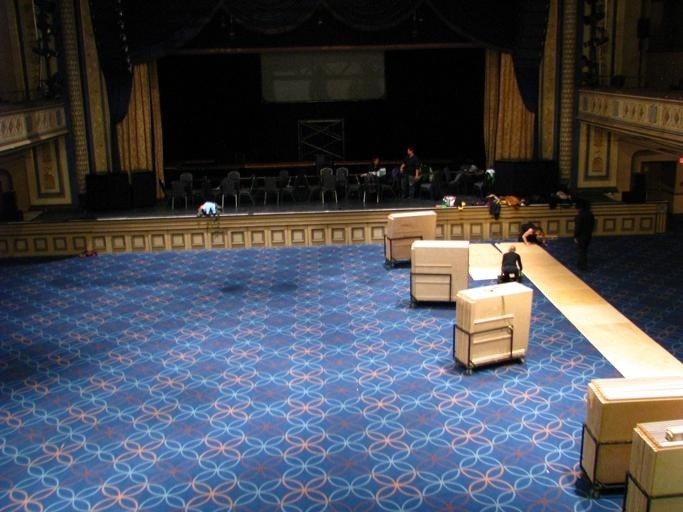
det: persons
[365,156,381,177]
[516,222,542,245]
[573,202,594,267]
[500,246,522,282]
[398,147,422,202]
